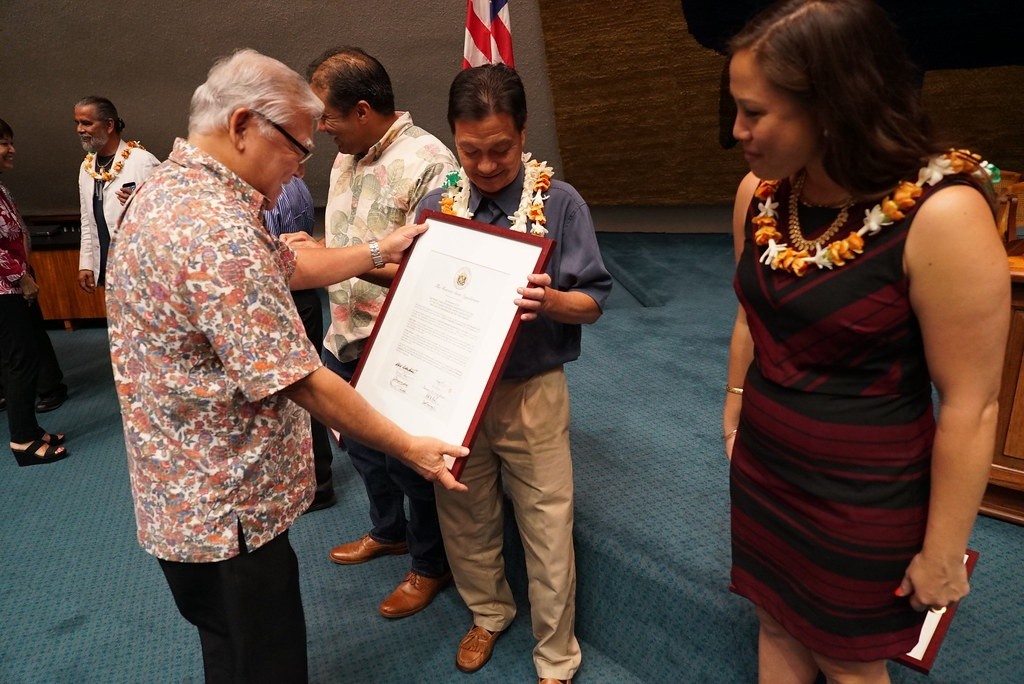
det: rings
[927,606,947,614]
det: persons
[259,174,337,514]
[103,48,470,684]
[411,63,614,684]
[721,2,1011,684]
[277,45,462,618]
[74,95,163,293]
[0,116,70,466]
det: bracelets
[721,430,738,438]
[725,384,744,394]
[368,239,384,270]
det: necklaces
[749,146,1000,280]
[437,150,555,237]
[81,142,147,182]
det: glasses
[252,109,316,165]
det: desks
[30,227,107,332]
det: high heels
[34,425,65,446]
[11,439,68,466]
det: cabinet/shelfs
[977,237,1024,523]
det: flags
[461,0,512,70]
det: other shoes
[35,391,68,413]
[304,491,338,515]
[0,398,7,411]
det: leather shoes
[456,623,500,672]
[329,532,408,564]
[538,672,571,684]
[380,563,452,618]
